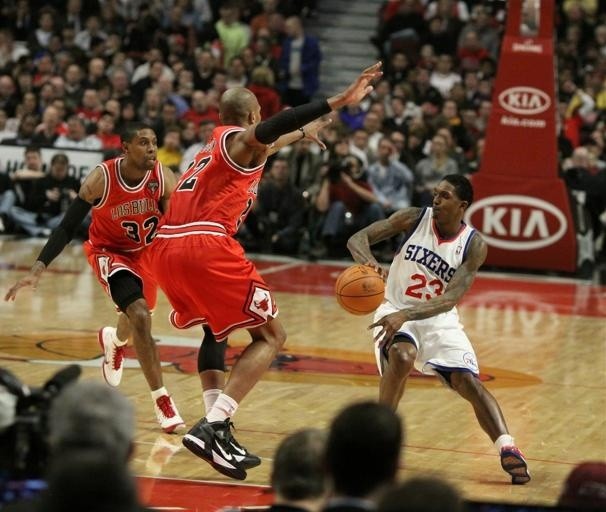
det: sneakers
[151,394,185,434]
[98,324,129,387]
[498,442,533,485]
[181,413,249,480]
[227,435,264,470]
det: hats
[50,153,69,167]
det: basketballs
[335,264,384,316]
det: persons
[0,1,606,281]
[369,474,463,510]
[343,170,529,489]
[263,427,333,510]
[553,459,606,512]
[145,58,385,482]
[38,379,149,509]
[316,399,404,510]
[0,120,191,434]
